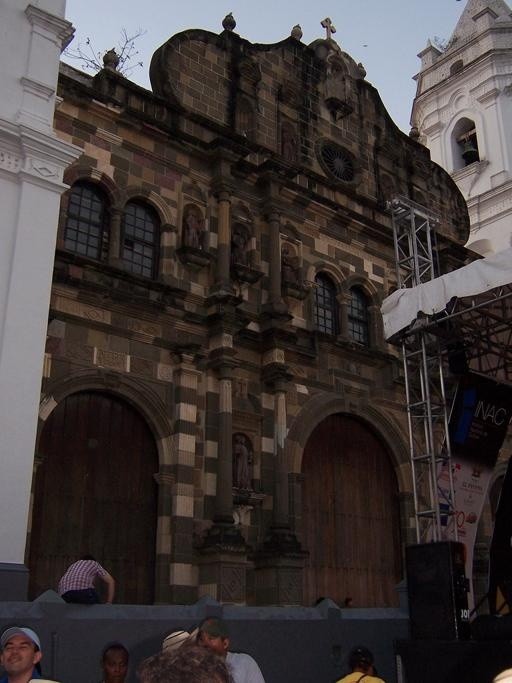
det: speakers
[405,540,471,641]
[440,369,512,468]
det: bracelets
[106,600,113,604]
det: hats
[0,626,41,649]
[201,619,226,637]
[348,646,374,666]
[162,627,199,649]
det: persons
[198,617,265,683]
[232,434,252,490]
[0,627,61,683]
[100,643,129,683]
[58,556,116,605]
[336,646,386,683]
